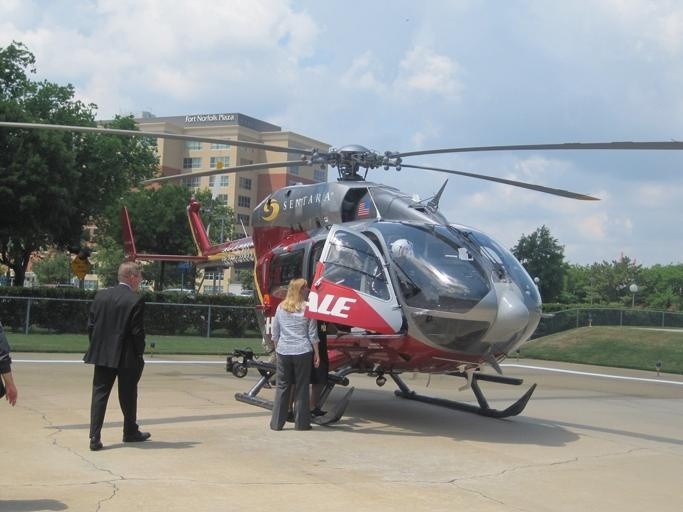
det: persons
[285,320,349,423]
[82,261,149,450]
[269,277,319,432]
[0,319,17,408]
[363,237,417,335]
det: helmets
[391,238,414,258]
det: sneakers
[286,407,328,423]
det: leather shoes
[88,436,105,451]
[121,430,152,443]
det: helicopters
[1,120,682,429]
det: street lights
[629,283,639,310]
[534,276,540,286]
[64,250,72,285]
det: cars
[35,284,261,332]
